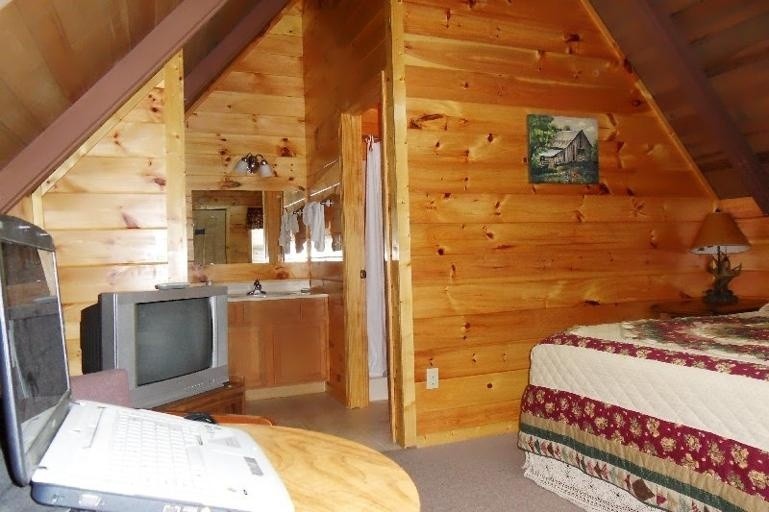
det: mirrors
[191,189,285,265]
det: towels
[276,184,342,253]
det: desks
[216,403,420,512]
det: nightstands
[654,298,767,315]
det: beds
[519,303,769,512]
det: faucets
[246,280,267,297]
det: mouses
[185,411,215,424]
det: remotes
[155,282,191,289]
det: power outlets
[427,366,439,390]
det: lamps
[235,153,274,178]
[689,208,748,304]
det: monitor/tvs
[79,285,231,409]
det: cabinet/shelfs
[228,296,330,400]
[149,375,246,420]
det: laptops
[0,212,296,512]
